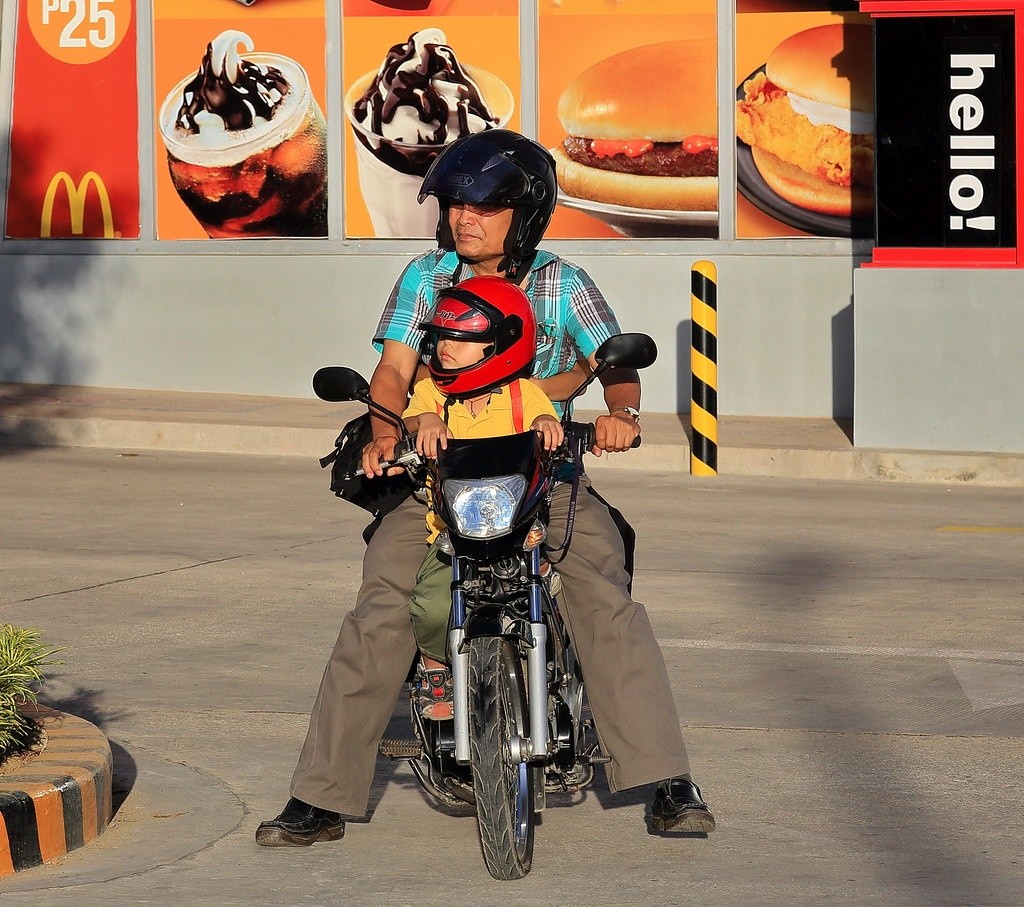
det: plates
[736,65,875,239]
[557,186,719,239]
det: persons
[399,275,564,721]
[255,129,715,845]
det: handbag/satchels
[319,412,427,517]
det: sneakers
[415,657,454,720]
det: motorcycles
[312,332,666,881]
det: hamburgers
[733,24,877,216]
[553,38,723,214]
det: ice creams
[164,29,304,165]
[354,27,499,238]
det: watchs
[610,406,641,425]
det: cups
[344,65,513,240]
[159,51,327,238]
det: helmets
[416,129,557,264]
[417,276,537,401]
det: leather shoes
[256,797,344,848]
[653,779,715,833]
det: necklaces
[466,394,491,419]
